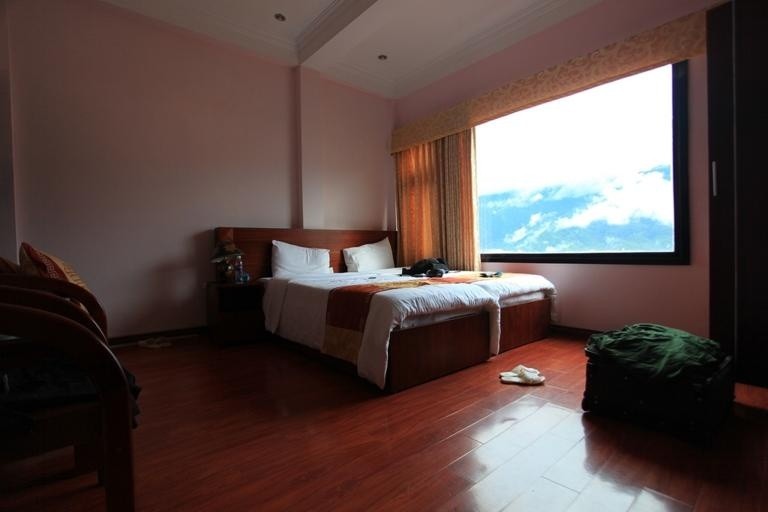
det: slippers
[499,365,540,377]
[501,369,545,384]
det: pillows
[0,242,91,294]
[343,236,395,272]
[272,239,330,278]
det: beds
[214,227,558,395]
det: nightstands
[207,282,263,349]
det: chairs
[0,278,142,512]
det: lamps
[209,240,245,282]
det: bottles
[235,255,242,282]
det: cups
[239,273,249,282]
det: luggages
[582,323,735,433]
